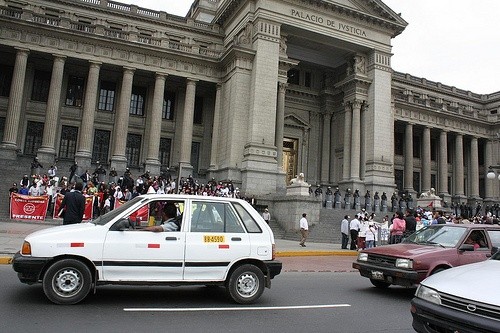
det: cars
[409,246,500,333]
[11,192,283,305]
[352,224,500,301]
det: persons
[134,170,257,219]
[8,183,19,220]
[354,189,500,250]
[349,214,360,250]
[334,186,341,208]
[309,187,314,194]
[298,213,308,247]
[324,186,332,207]
[343,188,351,209]
[314,185,323,196]
[20,157,134,217]
[262,208,270,226]
[340,215,349,249]
[57,183,86,226]
[140,204,180,232]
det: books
[57,209,65,218]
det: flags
[9,191,49,222]
[114,197,150,223]
[52,193,95,220]
